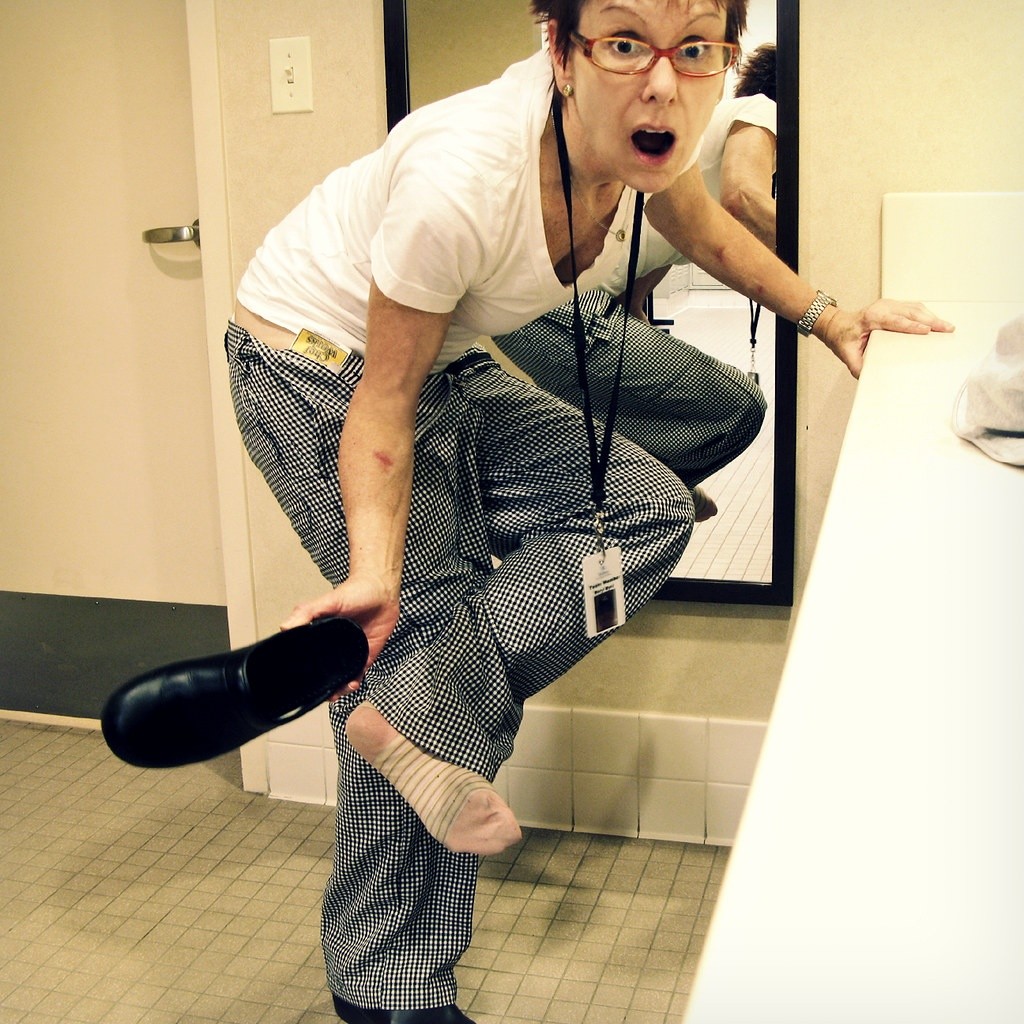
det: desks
[678,304,1024,1023]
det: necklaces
[571,175,633,242]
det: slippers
[333,993,477,1024]
[101,617,370,769]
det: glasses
[566,30,738,77]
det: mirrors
[379,0,799,610]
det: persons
[491,41,780,525]
[224,1,952,1023]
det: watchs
[796,290,837,337]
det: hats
[952,314,1024,467]
